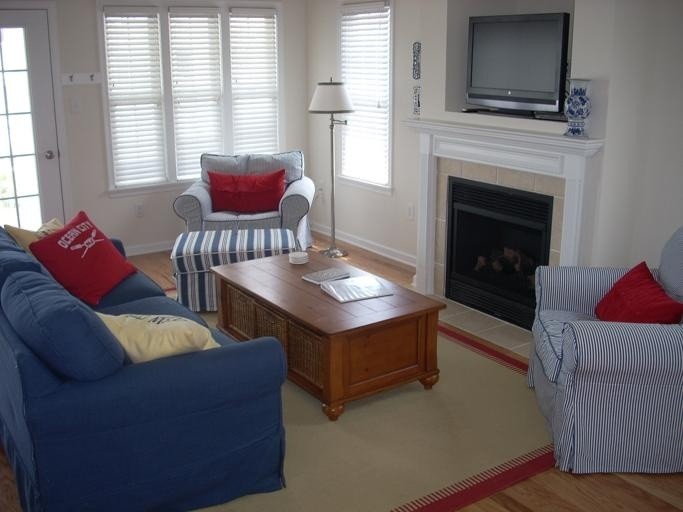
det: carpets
[165,285,558,511]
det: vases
[563,78,592,140]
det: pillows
[3,210,139,306]
[595,261,682,323]
[94,311,222,364]
[207,168,285,215]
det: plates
[289,251,309,265]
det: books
[318,272,393,304]
[300,266,348,286]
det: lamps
[308,77,356,259]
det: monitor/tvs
[462,11,572,122]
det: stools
[169,228,298,312]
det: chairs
[173,152,315,252]
[530,227,683,476]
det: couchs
[0,227,288,511]
